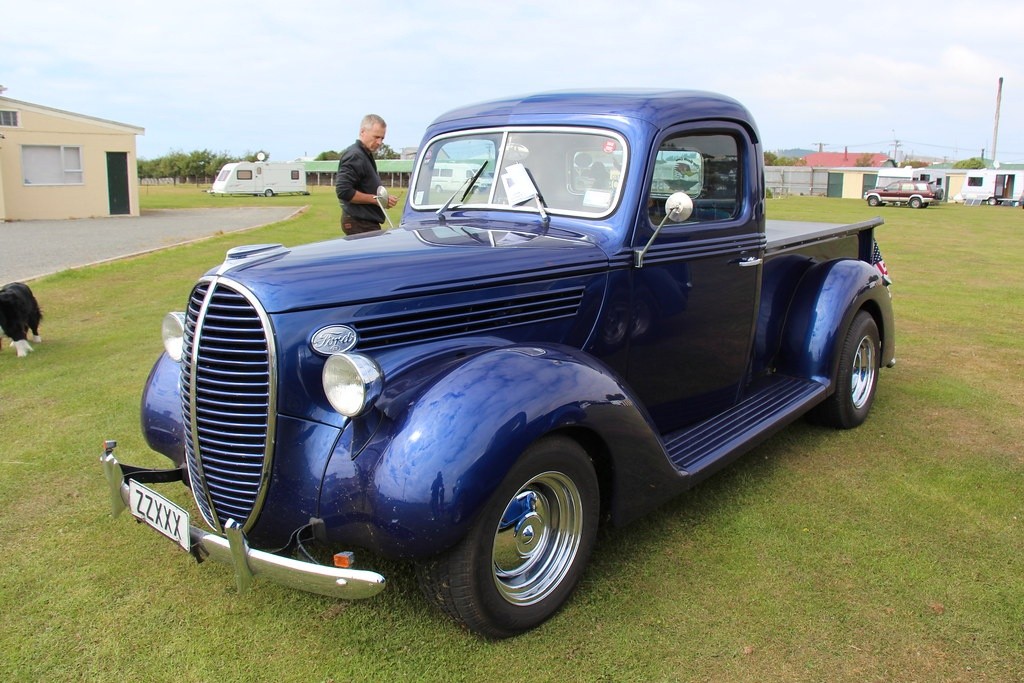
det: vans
[430,162,494,194]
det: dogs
[0,282,43,358]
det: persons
[335,113,397,236]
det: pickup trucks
[101,88,896,643]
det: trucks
[210,161,307,197]
[954,169,1023,205]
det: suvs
[863,180,944,208]
